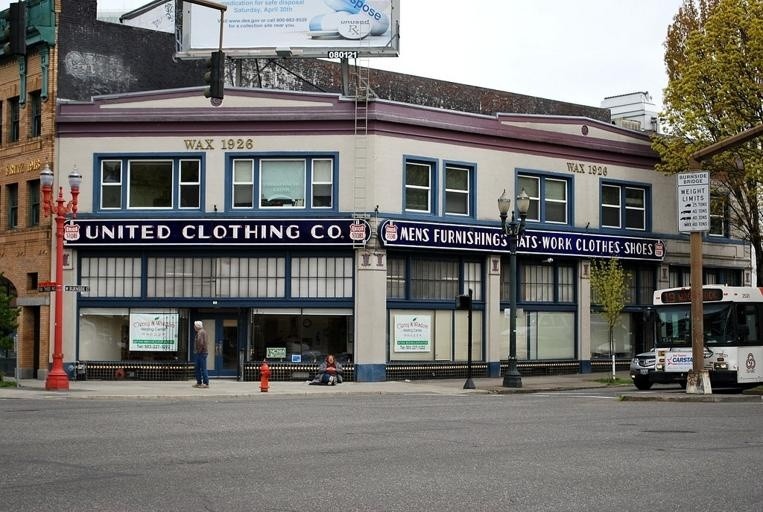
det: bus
[638,283,761,393]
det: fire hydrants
[257,363,271,393]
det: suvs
[597,331,630,356]
[630,345,688,390]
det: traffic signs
[39,286,57,291]
[64,285,90,292]
[675,170,712,233]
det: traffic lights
[1,3,19,59]
[199,47,221,98]
[453,293,472,309]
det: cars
[503,300,575,348]
[273,340,320,363]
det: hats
[195,321,203,328]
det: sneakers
[193,384,209,387]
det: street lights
[493,186,534,389]
[30,163,86,397]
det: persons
[318,354,343,386]
[191,321,209,388]
